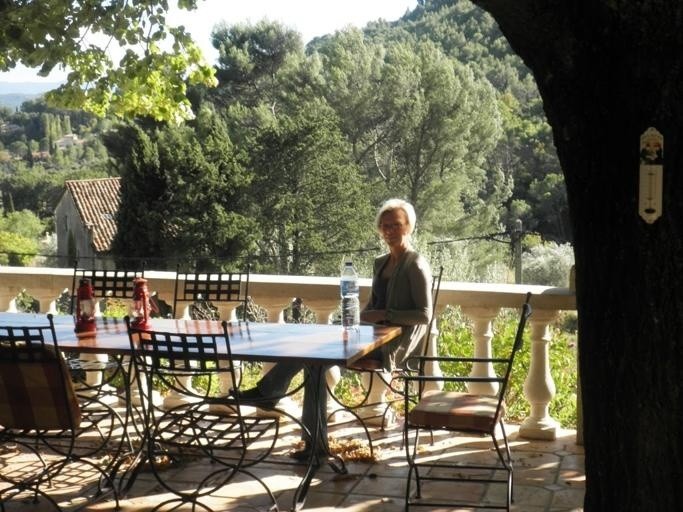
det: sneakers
[284,449,329,461]
[228,384,275,413]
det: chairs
[0,314,113,509]
[390,290,533,512]
[317,264,445,451]
[64,254,145,411]
[137,256,256,411]
[121,314,282,511]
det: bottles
[340,261,361,332]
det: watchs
[382,307,396,325]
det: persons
[228,197,435,462]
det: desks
[0,312,403,511]
